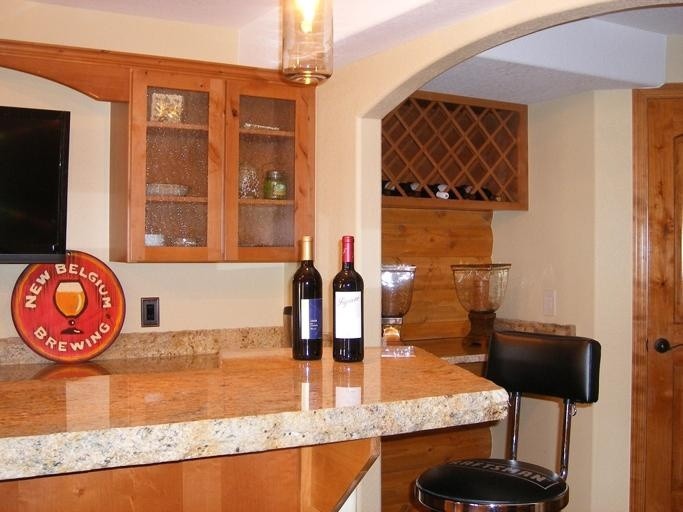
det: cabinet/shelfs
[108,65,314,261]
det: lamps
[279,1,334,87]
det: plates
[145,183,189,197]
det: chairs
[414,331,602,510]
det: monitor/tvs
[0,105,72,263]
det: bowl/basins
[145,235,166,248]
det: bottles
[293,237,323,359]
[333,235,364,363]
[381,179,502,202]
[239,160,290,200]
